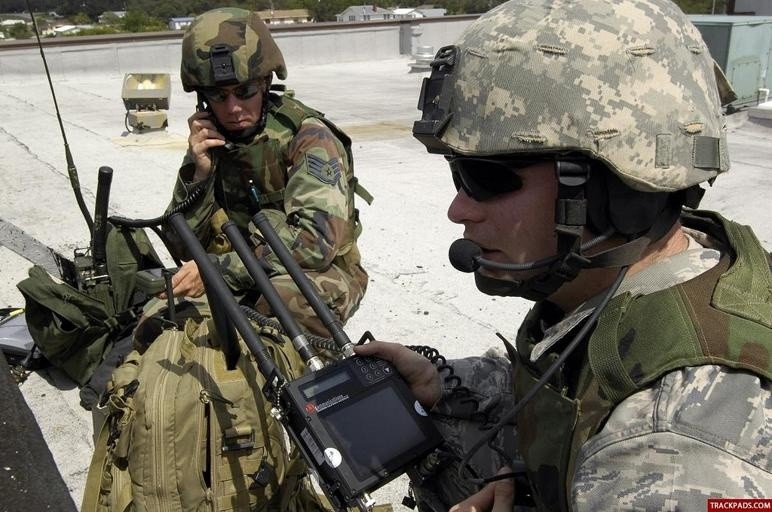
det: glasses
[438,149,568,207]
[201,81,269,102]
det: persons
[153,7,368,338]
[353,0,770,512]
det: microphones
[449,228,616,273]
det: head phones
[589,163,670,237]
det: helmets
[179,8,287,92]
[413,1,732,192]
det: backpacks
[14,215,167,384]
[81,304,318,512]
